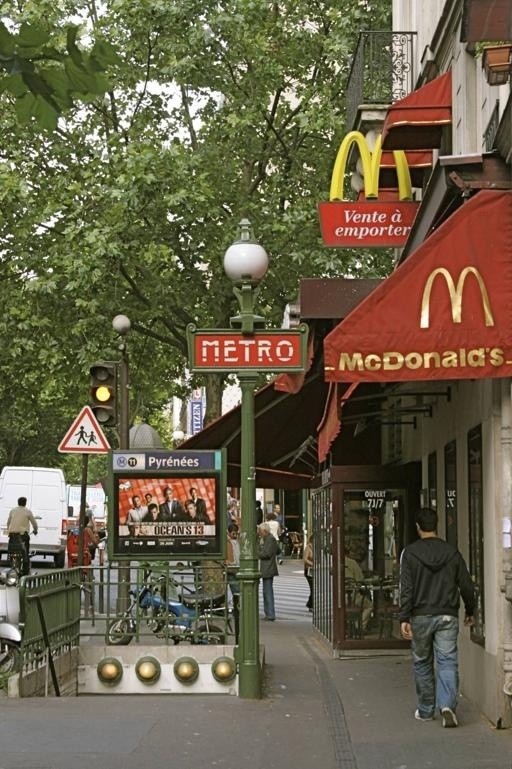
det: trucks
[67,485,107,540]
[0,466,73,569]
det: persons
[397,504,479,728]
[6,497,38,575]
[344,546,373,634]
[224,492,285,621]
[124,486,210,524]
[76,501,108,564]
[302,534,313,612]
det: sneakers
[414,708,435,723]
[440,707,458,730]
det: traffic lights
[90,364,115,427]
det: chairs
[343,575,399,640]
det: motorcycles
[0,569,25,690]
[10,531,36,587]
[106,562,232,645]
[73,531,106,604]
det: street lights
[225,218,269,702]
[112,314,131,612]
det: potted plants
[476,41,511,86]
